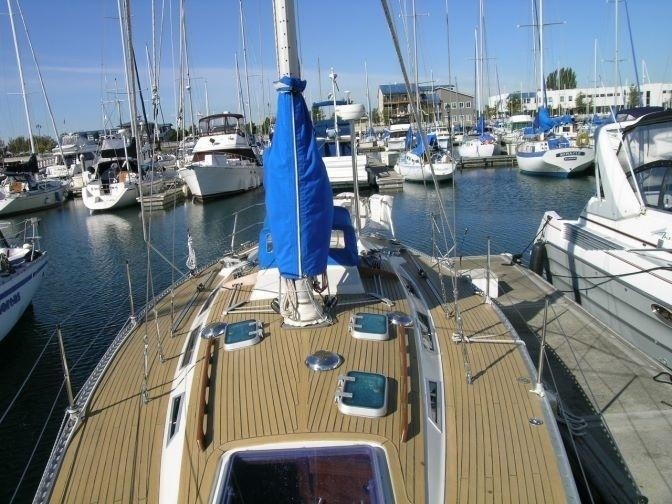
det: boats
[532,106,672,368]
[2,216,50,335]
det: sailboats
[0,1,76,213]
[45,0,275,214]
[322,0,649,195]
[29,0,584,504]
